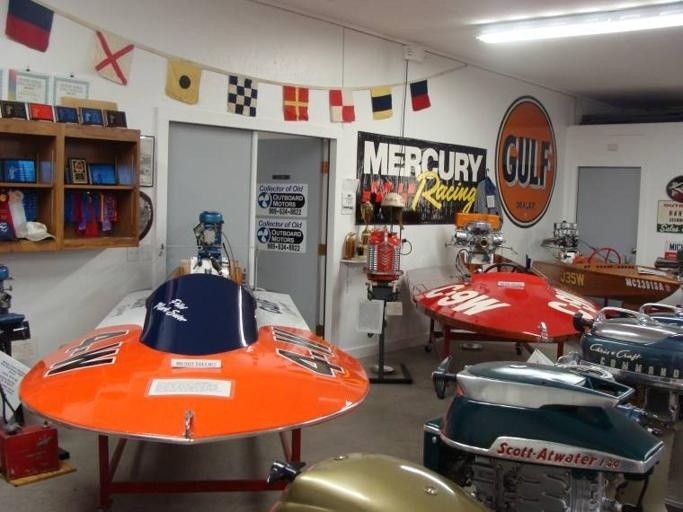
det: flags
[4,0,55,53]
[369,86,394,121]
[327,89,356,125]
[90,28,136,88]
[409,80,430,112]
[163,55,203,105]
[224,75,260,118]
[281,85,309,121]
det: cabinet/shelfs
[0,117,140,252]
